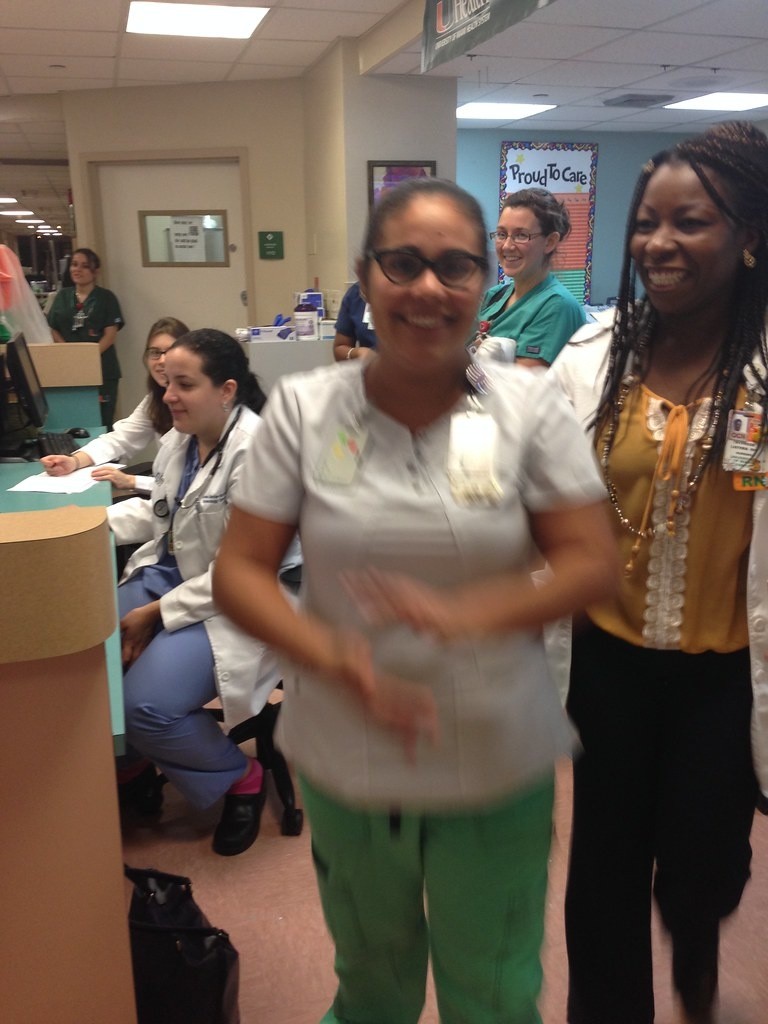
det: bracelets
[64,453,80,471]
[345,348,355,359]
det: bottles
[292,292,320,343]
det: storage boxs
[320,319,337,340]
[293,290,324,308]
[317,308,325,318]
[247,324,297,342]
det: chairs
[132,562,303,838]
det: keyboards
[38,432,80,456]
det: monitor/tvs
[5,332,50,427]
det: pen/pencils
[196,502,204,521]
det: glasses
[490,231,542,243]
[365,250,487,285]
[150,350,166,360]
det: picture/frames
[366,159,437,213]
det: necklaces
[600,306,732,539]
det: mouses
[67,428,90,438]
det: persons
[46,248,125,439]
[40,316,303,858]
[332,280,377,363]
[464,188,590,368]
[543,121,768,1024]
[213,176,624,1024]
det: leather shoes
[213,758,267,855]
[127,766,162,819]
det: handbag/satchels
[124,863,239,1024]
